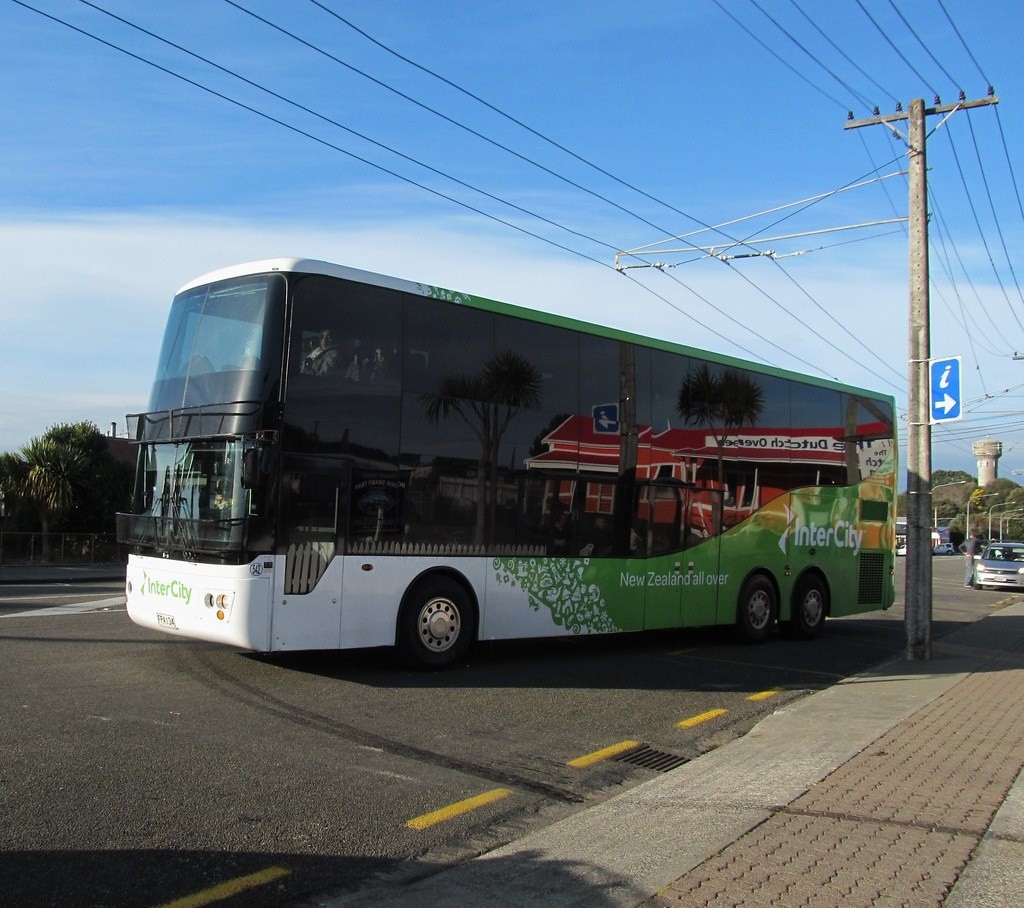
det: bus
[895,521,906,556]
[115,255,900,672]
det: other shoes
[964,584,974,588]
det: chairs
[344,349,430,395]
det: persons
[304,327,385,383]
[958,532,998,588]
[541,516,611,557]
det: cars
[932,542,956,555]
[972,542,1024,592]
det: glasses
[214,487,231,492]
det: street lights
[999,508,1024,543]
[966,492,1000,541]
[1006,513,1023,535]
[988,502,1016,544]
